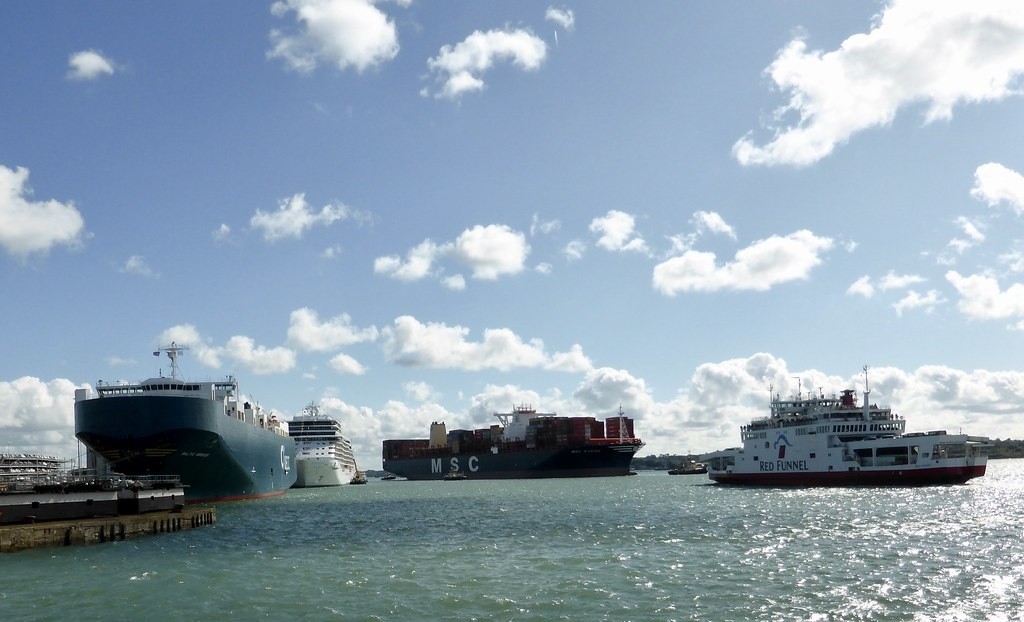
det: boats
[350,459,369,484]
[380,474,396,480]
[668,461,708,474]
[382,401,646,479]
[708,364,994,489]
[287,401,355,488]
[1,440,192,526]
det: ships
[72,341,299,504]
